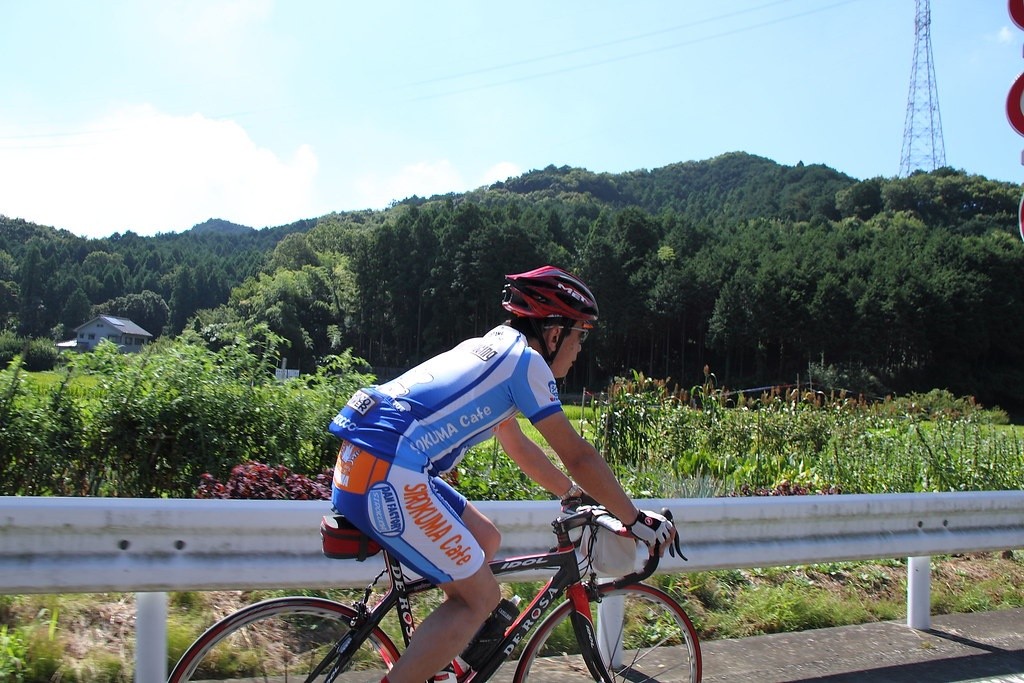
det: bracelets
[556,480,578,499]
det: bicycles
[167,493,704,683]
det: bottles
[459,594,522,673]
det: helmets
[502,265,599,322]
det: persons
[326,264,676,683]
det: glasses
[543,326,590,345]
